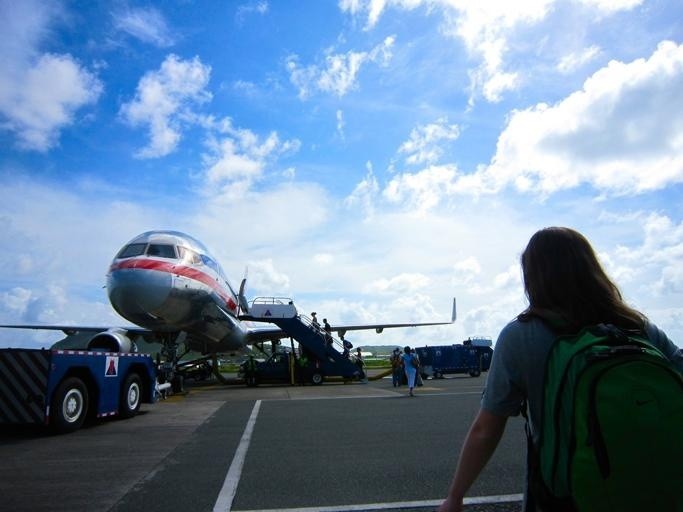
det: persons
[288,300,298,318]
[309,311,318,332]
[433,225,681,509]
[321,318,333,347]
[389,345,424,398]
[339,334,353,359]
[353,346,363,371]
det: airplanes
[0,226,458,388]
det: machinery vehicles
[234,350,352,383]
[414,336,495,381]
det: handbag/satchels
[409,355,420,369]
[416,371,424,387]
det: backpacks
[528,303,683,512]
